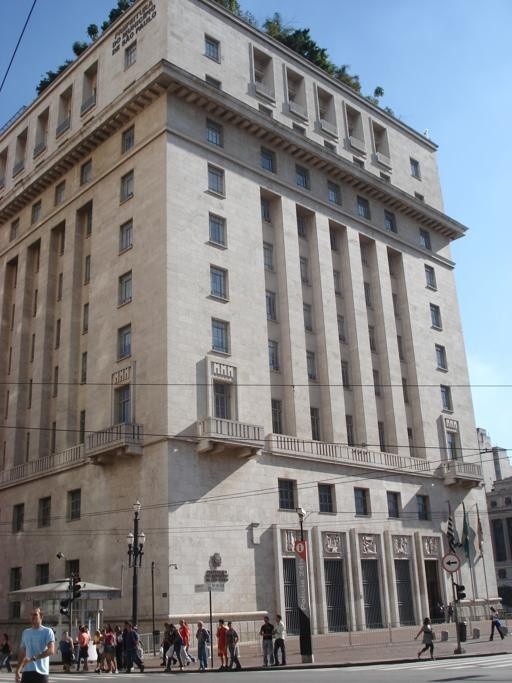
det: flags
[474,509,485,563]
[461,501,474,566]
[446,503,457,555]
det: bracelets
[33,655,37,661]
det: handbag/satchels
[496,619,501,626]
[430,632,436,640]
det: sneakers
[161,661,167,667]
[173,659,177,665]
[62,665,145,674]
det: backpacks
[165,644,175,658]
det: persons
[259,616,274,667]
[271,615,287,666]
[160,619,196,671]
[415,618,436,661]
[437,601,455,624]
[206,629,211,658]
[0,633,13,673]
[489,606,504,641]
[216,620,229,672]
[59,620,144,673]
[196,621,209,671]
[15,606,55,683]
[224,620,241,672]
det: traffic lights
[72,574,82,598]
[60,595,71,616]
[456,584,466,601]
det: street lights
[126,500,146,639]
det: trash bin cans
[460,622,466,642]
